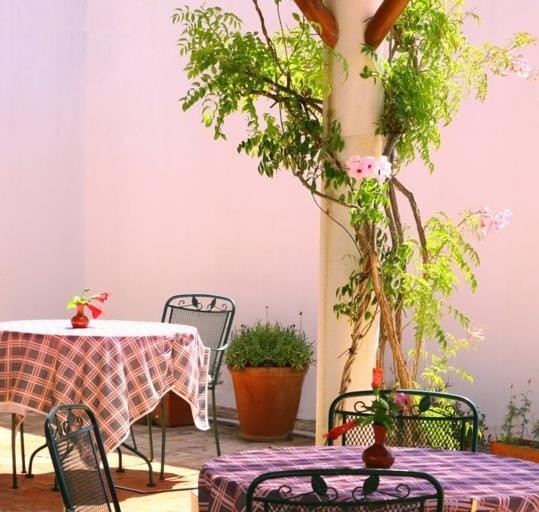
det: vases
[147,392,193,427]
[363,424,394,468]
[70,305,89,328]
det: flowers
[64,287,108,319]
[355,367,411,434]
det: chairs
[116,294,236,480]
[246,468,444,512]
[328,390,479,452]
[44,404,121,512]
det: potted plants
[491,377,539,463]
[224,320,315,443]
